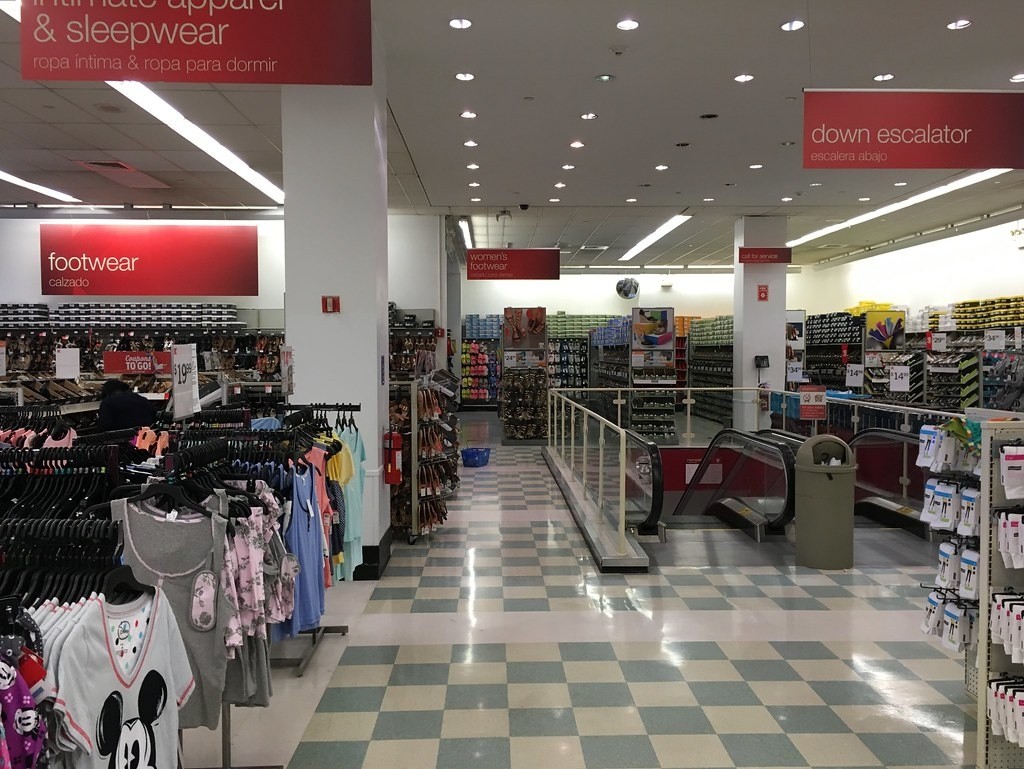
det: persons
[505,308,546,342]
[99,380,155,429]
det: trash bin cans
[795,433,857,569]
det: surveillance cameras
[519,204,529,210]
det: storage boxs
[928,294,1023,330]
[464,310,733,344]
[805,312,861,344]
[0,303,245,331]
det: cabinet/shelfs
[585,328,1023,444]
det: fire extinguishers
[757,380,772,411]
[384,423,404,484]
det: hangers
[0,402,359,608]
